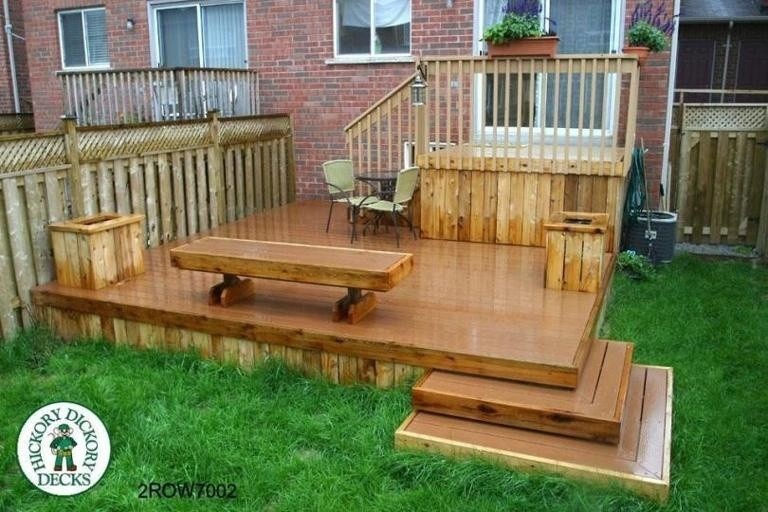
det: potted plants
[481,10,559,56]
[619,23,665,64]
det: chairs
[322,159,420,248]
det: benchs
[169,237,414,330]
